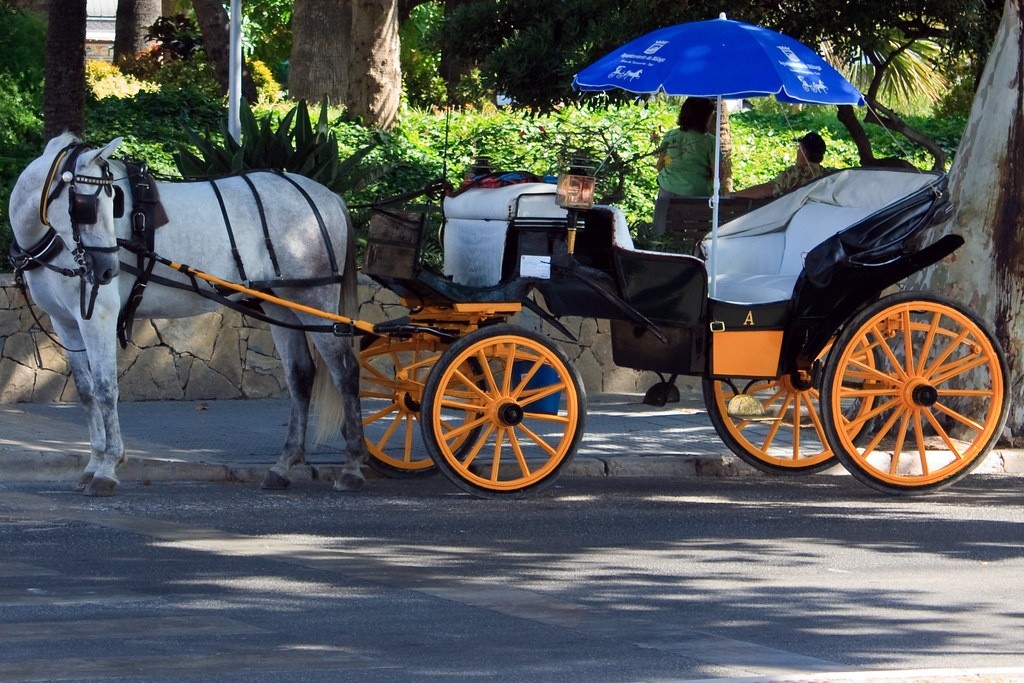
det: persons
[651,95,725,236]
[723,131,841,199]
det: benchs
[665,196,768,240]
[575,203,708,321]
[702,200,874,305]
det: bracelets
[732,192,735,198]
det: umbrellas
[571,11,866,297]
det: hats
[791,132,826,153]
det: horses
[9,125,370,496]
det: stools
[443,181,569,222]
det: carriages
[9,13,1012,497]
